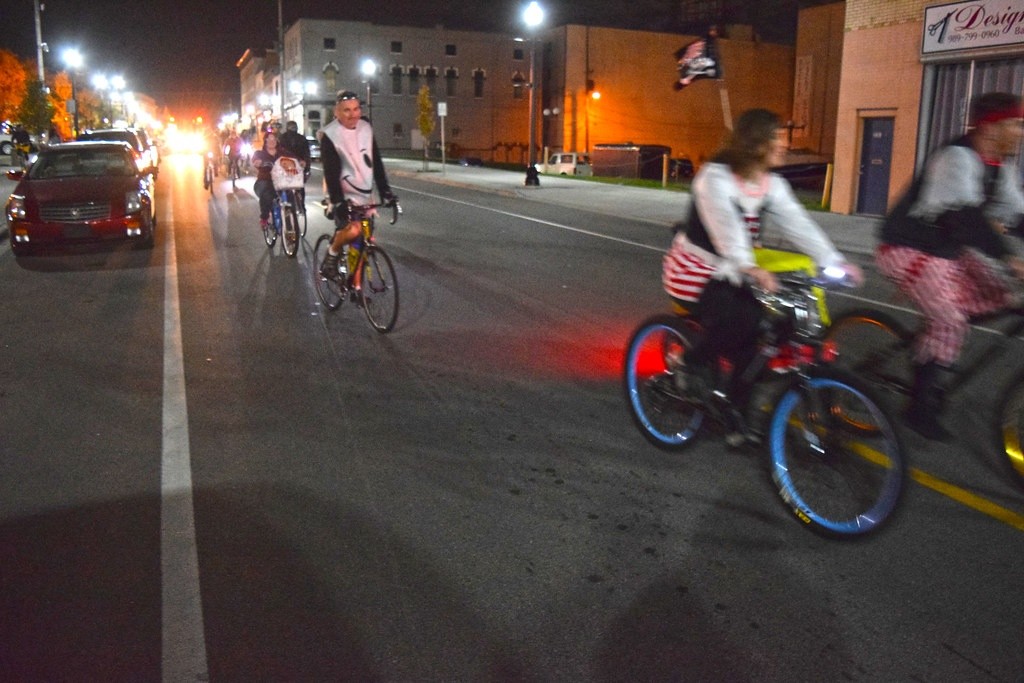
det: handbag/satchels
[271,156,304,190]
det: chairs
[57,156,80,175]
[106,155,124,173]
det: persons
[874,91,1024,443]
[12,123,30,160]
[200,127,244,189]
[251,121,311,226]
[49,127,61,144]
[662,108,862,456]
[316,91,392,305]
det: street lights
[523,3,544,188]
[361,59,377,122]
[64,49,84,131]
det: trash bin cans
[11,148,23,167]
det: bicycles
[816,269,1024,489]
[313,199,399,334]
[624,271,904,542]
[204,148,309,258]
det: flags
[673,34,723,92]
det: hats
[968,91,1024,127]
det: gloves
[383,191,395,201]
[335,201,348,218]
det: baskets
[271,170,305,190]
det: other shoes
[320,251,339,277]
[350,289,372,306]
[663,352,699,401]
[900,406,951,444]
[261,218,268,231]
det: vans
[534,152,593,177]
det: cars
[308,138,322,160]
[76,124,166,164]
[0,122,19,154]
[2,141,156,255]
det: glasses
[338,95,358,101]
